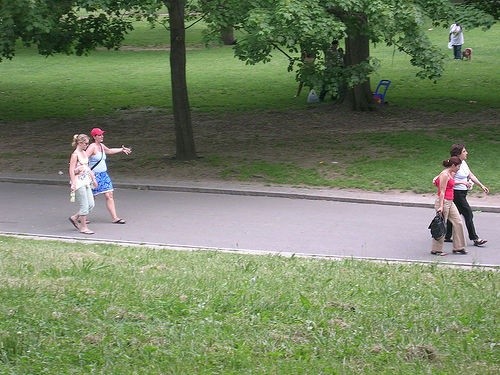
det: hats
[90,128,106,136]
[448,42,453,49]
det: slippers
[78,220,90,223]
[112,219,125,223]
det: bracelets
[92,178,95,181]
[479,184,484,188]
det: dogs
[462,48,472,61]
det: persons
[85,128,132,224]
[68,134,98,235]
[430,156,468,256]
[294,51,316,98]
[449,21,464,60]
[444,144,489,246]
[319,40,345,103]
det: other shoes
[69,216,79,229]
[80,229,94,234]
[431,251,447,256]
[453,248,468,254]
[444,238,453,241]
[474,240,487,245]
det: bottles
[467,178,473,190]
[70,191,76,203]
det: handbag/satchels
[428,210,445,240]
[433,173,440,186]
[308,89,319,103]
